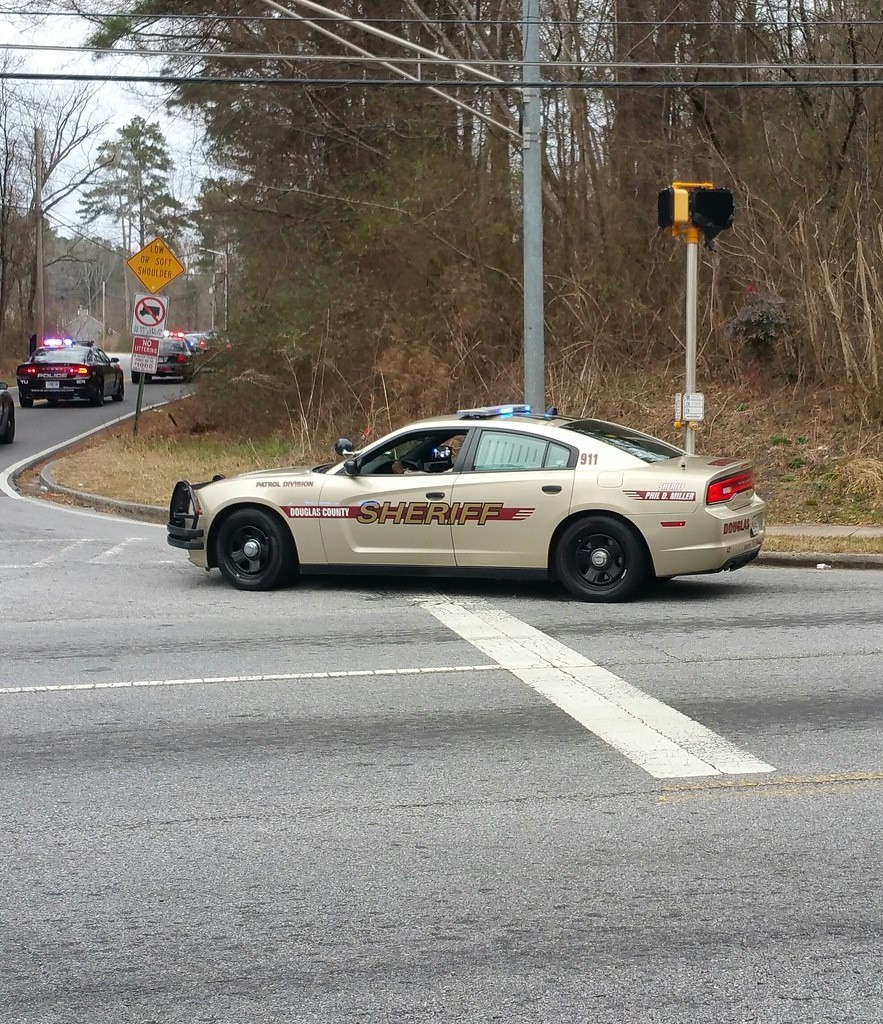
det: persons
[392,461,454,474]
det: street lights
[200,247,229,332]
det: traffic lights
[658,187,689,228]
[688,187,736,234]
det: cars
[167,403,766,604]
[0,382,15,445]
[131,331,197,382]
[184,332,231,371]
[17,339,125,407]
